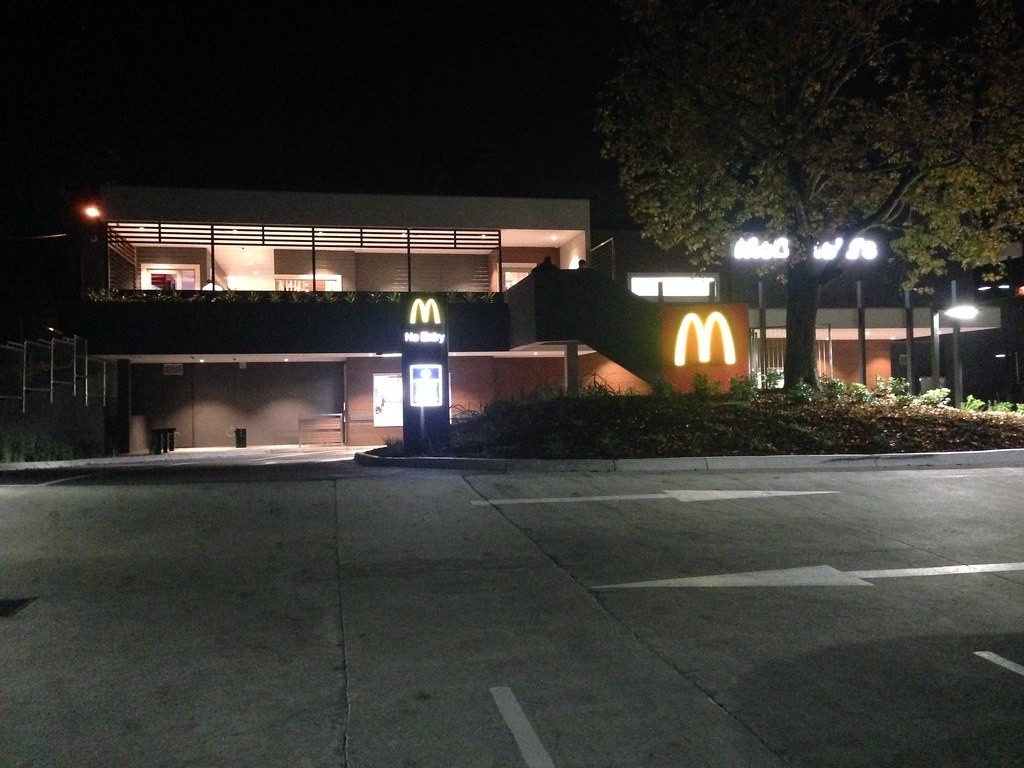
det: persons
[201,280,223,291]
[578,260,587,269]
[538,256,560,270]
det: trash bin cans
[235,428,246,448]
[151,427,178,454]
